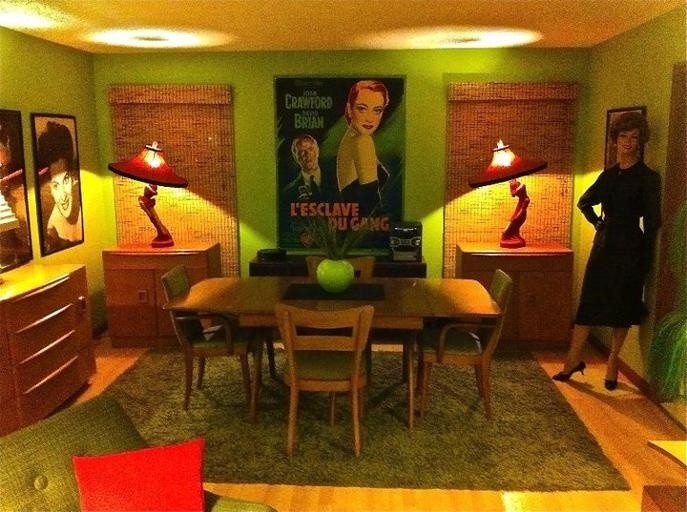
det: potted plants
[299,213,373,293]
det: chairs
[160,261,254,411]
[305,254,376,278]
[273,301,369,460]
[404,269,511,420]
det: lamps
[469,137,547,248]
[109,139,187,247]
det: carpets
[102,345,632,490]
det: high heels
[605,380,617,391]
[553,361,585,381]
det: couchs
[2,393,276,512]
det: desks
[160,276,504,431]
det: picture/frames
[603,106,647,171]
[273,73,407,253]
[29,110,84,257]
[0,109,33,274]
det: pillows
[72,435,208,512]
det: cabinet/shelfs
[250,254,428,281]
[101,241,221,348]
[454,242,575,353]
[1,262,96,438]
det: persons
[334,78,393,217]
[282,134,336,203]
[36,121,84,250]
[549,110,662,393]
